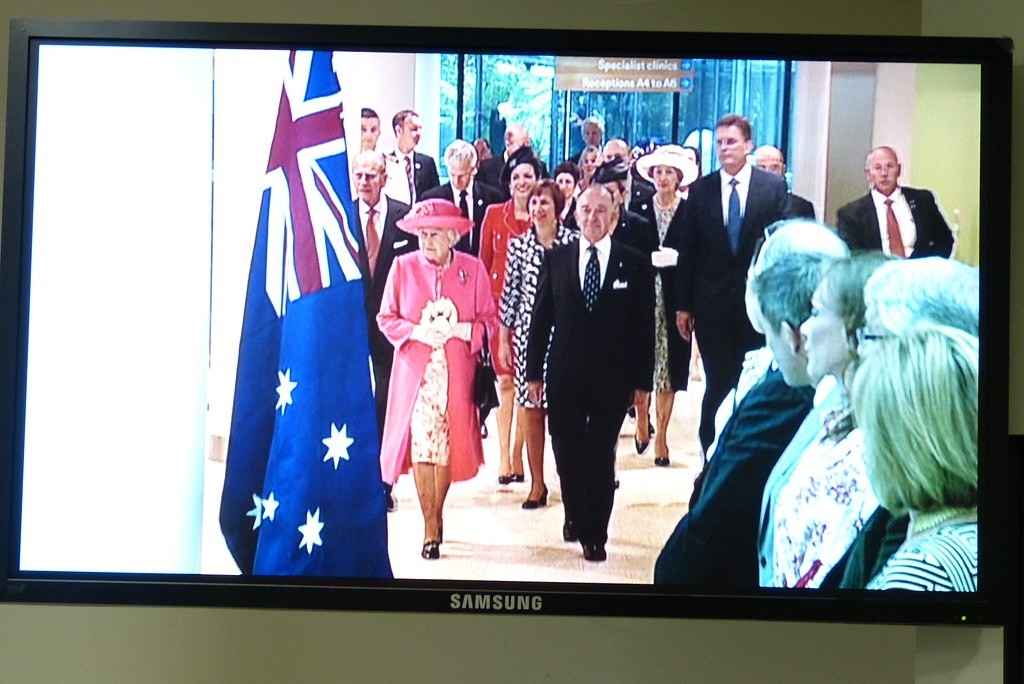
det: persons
[835,146,955,258]
[526,184,656,563]
[353,107,603,510]
[375,200,498,558]
[683,147,704,180]
[635,143,700,465]
[658,114,794,457]
[754,147,814,219]
[650,218,978,593]
[600,139,655,434]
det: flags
[218,51,389,577]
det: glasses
[751,218,786,267]
[852,329,890,355]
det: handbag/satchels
[471,321,499,438]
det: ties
[458,190,470,253]
[884,199,906,259]
[728,178,742,255]
[364,209,380,278]
[583,246,601,313]
[403,156,413,209]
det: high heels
[523,486,548,509]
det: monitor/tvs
[0,19,1014,625]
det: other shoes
[654,446,670,466]
[647,414,655,434]
[634,433,650,454]
[422,526,443,559]
[384,490,393,509]
[498,472,516,484]
[514,474,525,483]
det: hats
[635,144,699,186]
[595,157,628,183]
[396,199,475,238]
[498,146,539,188]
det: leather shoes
[579,536,607,561]
[563,518,580,541]
